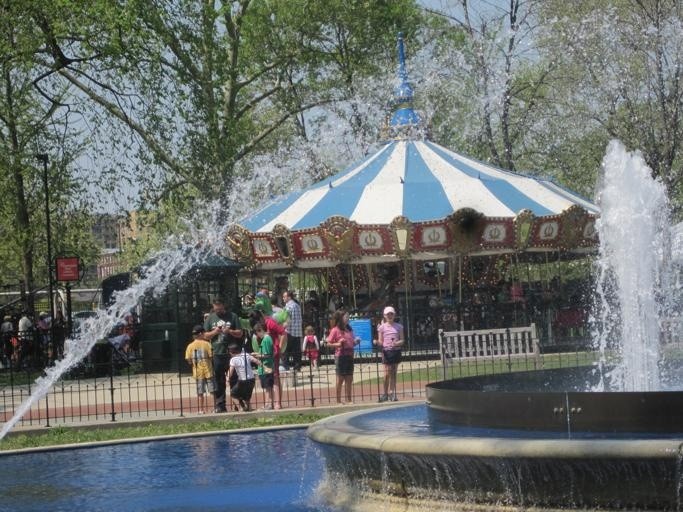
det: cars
[64,311,96,328]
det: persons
[497,278,524,304]
[185,289,405,414]
[0,310,66,372]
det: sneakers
[212,407,221,413]
[261,404,274,410]
[199,410,206,414]
[389,392,398,401]
[337,400,354,406]
[379,395,388,402]
[232,405,252,412]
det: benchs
[437,323,545,383]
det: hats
[39,312,48,317]
[4,315,11,320]
[383,307,395,315]
[213,297,224,304]
[192,325,205,335]
